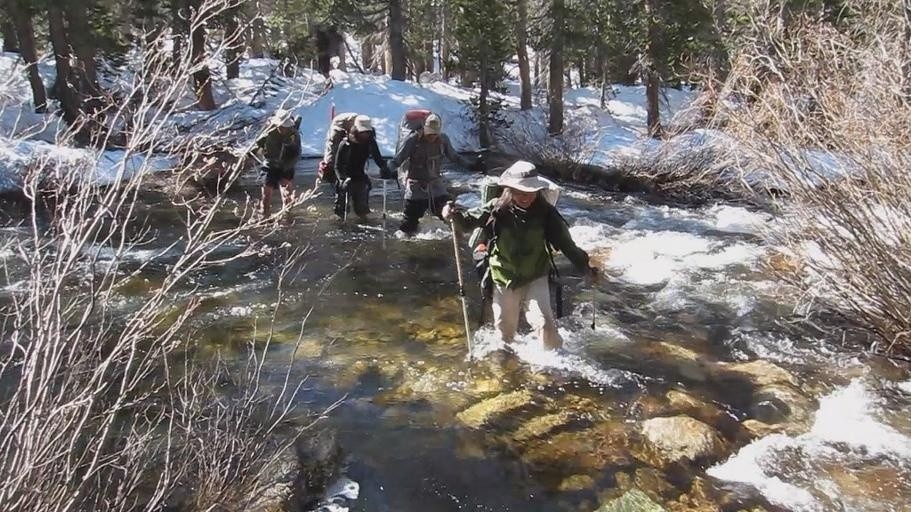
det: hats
[354,115,372,133]
[498,160,549,192]
[272,110,295,127]
[424,114,441,135]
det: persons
[382,114,487,240]
[443,160,598,352]
[260,108,302,226]
[332,113,393,222]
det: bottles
[473,244,486,260]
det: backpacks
[318,112,359,183]
[469,176,561,302]
[395,110,432,189]
